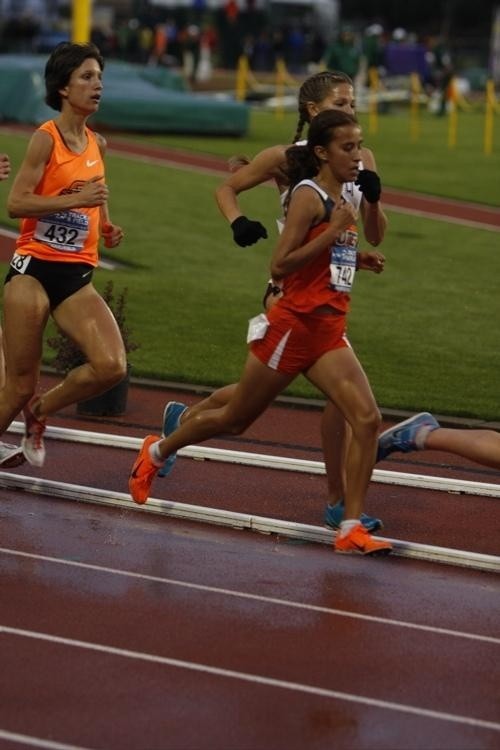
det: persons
[158,69,387,534]
[0,0,70,52]
[1,40,132,472]
[1,149,26,470]
[92,0,453,86]
[125,106,394,555]
[376,406,500,478]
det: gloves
[354,170,382,203]
[230,216,268,248]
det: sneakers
[20,395,46,467]
[334,522,394,556]
[128,435,165,505]
[158,401,190,479]
[373,412,440,466]
[0,440,27,468]
[323,498,384,533]
